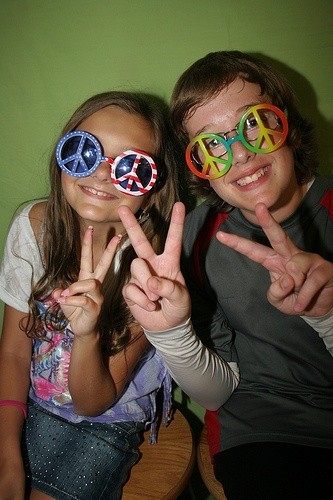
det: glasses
[191,110,280,165]
[55,130,158,197]
[185,104,289,179]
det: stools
[119,404,227,500]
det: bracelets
[0,400,28,419]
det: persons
[0,92,180,500]
[117,51,333,500]
